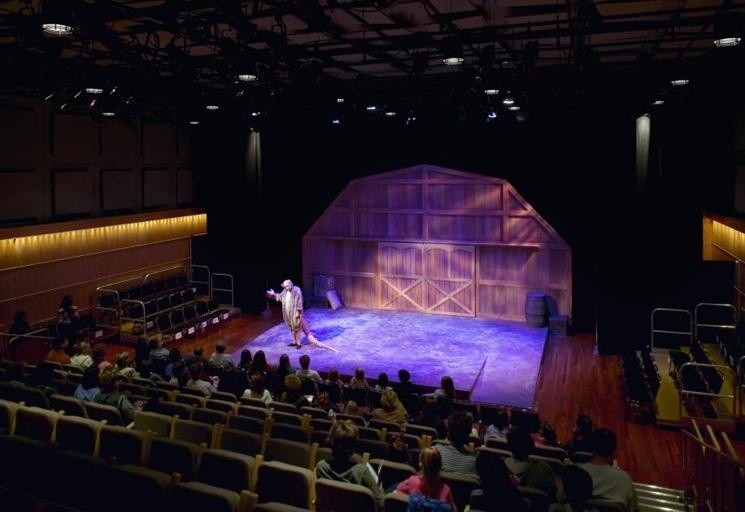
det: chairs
[101,273,229,348]
[0,359,592,512]
[622,324,745,422]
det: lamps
[38,9,81,36]
[38,80,136,119]
[470,45,542,127]
[186,81,274,126]
[331,92,419,128]
[440,37,465,67]
[236,62,259,82]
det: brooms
[592,314,599,356]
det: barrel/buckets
[524,291,547,328]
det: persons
[57,296,80,326]
[266,278,340,353]
[8,309,32,335]
[47,335,637,511]
